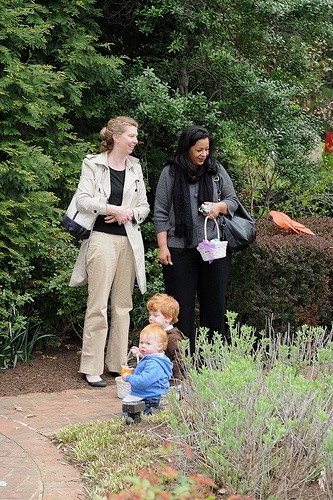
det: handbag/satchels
[215,169,256,254]
[62,157,101,240]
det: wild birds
[269,211,315,235]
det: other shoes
[109,372,121,377]
[84,373,106,387]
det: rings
[212,217,214,218]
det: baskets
[196,215,228,263]
[116,348,141,399]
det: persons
[122,293,185,417]
[69,116,151,386]
[154,126,238,371]
